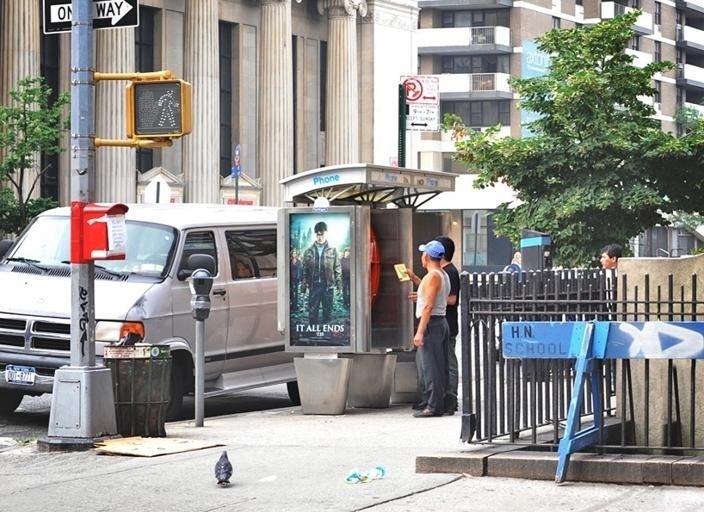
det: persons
[510,252,523,269]
[598,244,622,275]
[408,237,460,411]
[290,221,350,327]
[402,241,451,418]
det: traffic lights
[125,80,192,137]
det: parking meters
[188,267,213,428]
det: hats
[418,241,445,259]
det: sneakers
[411,399,458,417]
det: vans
[1,203,300,422]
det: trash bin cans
[103,344,173,438]
